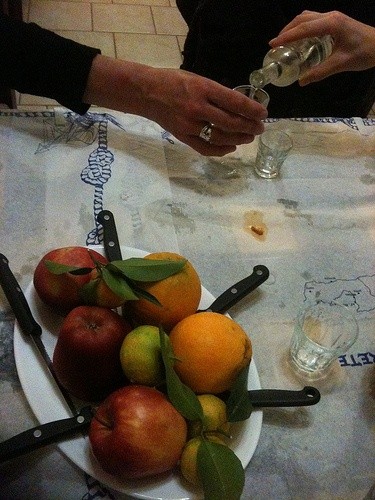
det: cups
[233,85,270,108]
[287,297,359,381]
[253,129,296,179]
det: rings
[199,123,214,142]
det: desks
[0,111,375,500]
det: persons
[0,11,268,157]
[176,0,375,118]
[270,11,375,86]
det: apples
[33,246,110,310]
[87,386,187,480]
[52,305,132,405]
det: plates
[12,242,264,500]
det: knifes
[0,253,88,440]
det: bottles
[249,33,335,87]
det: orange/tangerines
[167,312,253,394]
[90,275,127,307]
[125,252,201,331]
[121,325,228,488]
